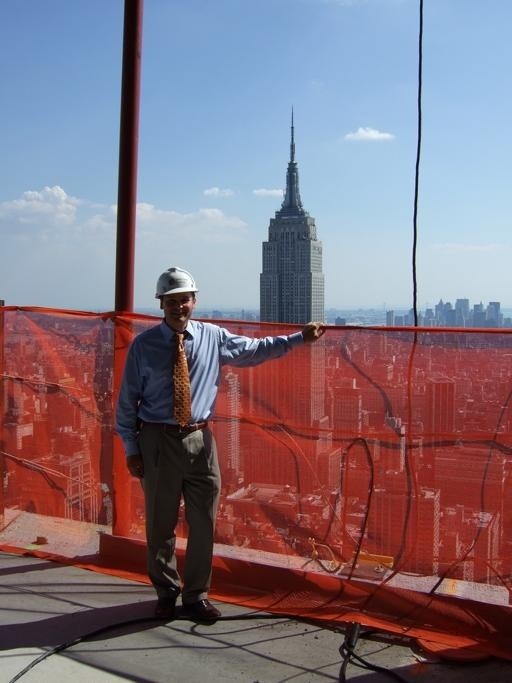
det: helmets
[155,266,199,298]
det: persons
[114,263,325,619]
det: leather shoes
[178,599,221,619]
[155,596,180,620]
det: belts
[137,420,208,434]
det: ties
[172,333,193,428]
